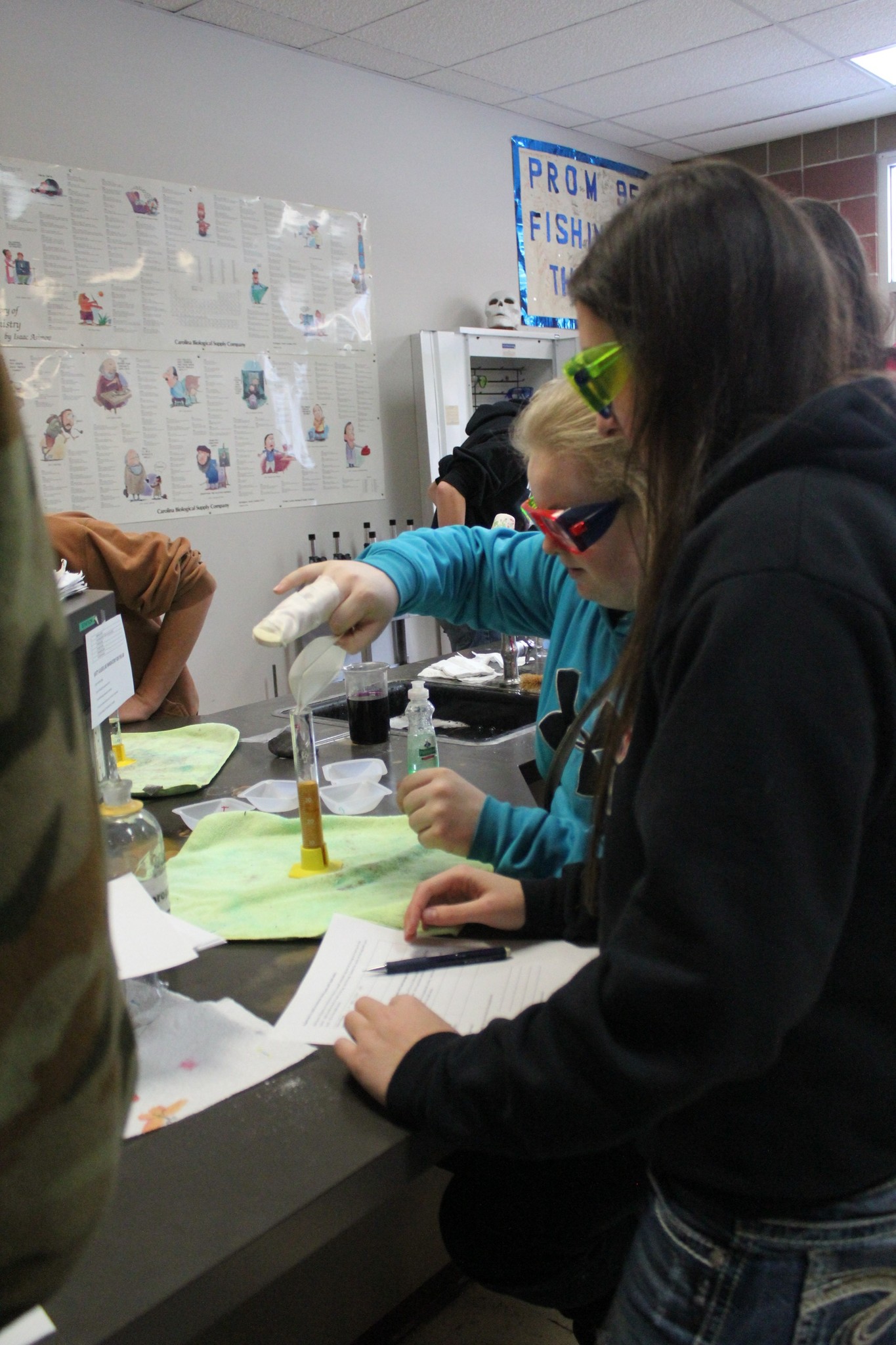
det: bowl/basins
[236,780,299,812]
[318,759,393,814]
[171,797,256,831]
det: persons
[790,192,895,377]
[0,360,137,1332]
[251,373,653,886]
[424,395,534,652]
[38,507,221,725]
[338,159,895,1345]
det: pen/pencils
[365,947,513,974]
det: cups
[341,661,390,744]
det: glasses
[564,339,647,414]
[518,490,641,556]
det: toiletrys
[404,680,439,776]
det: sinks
[272,677,545,745]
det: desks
[18,685,612,1344]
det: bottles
[97,752,173,915]
[404,682,441,775]
